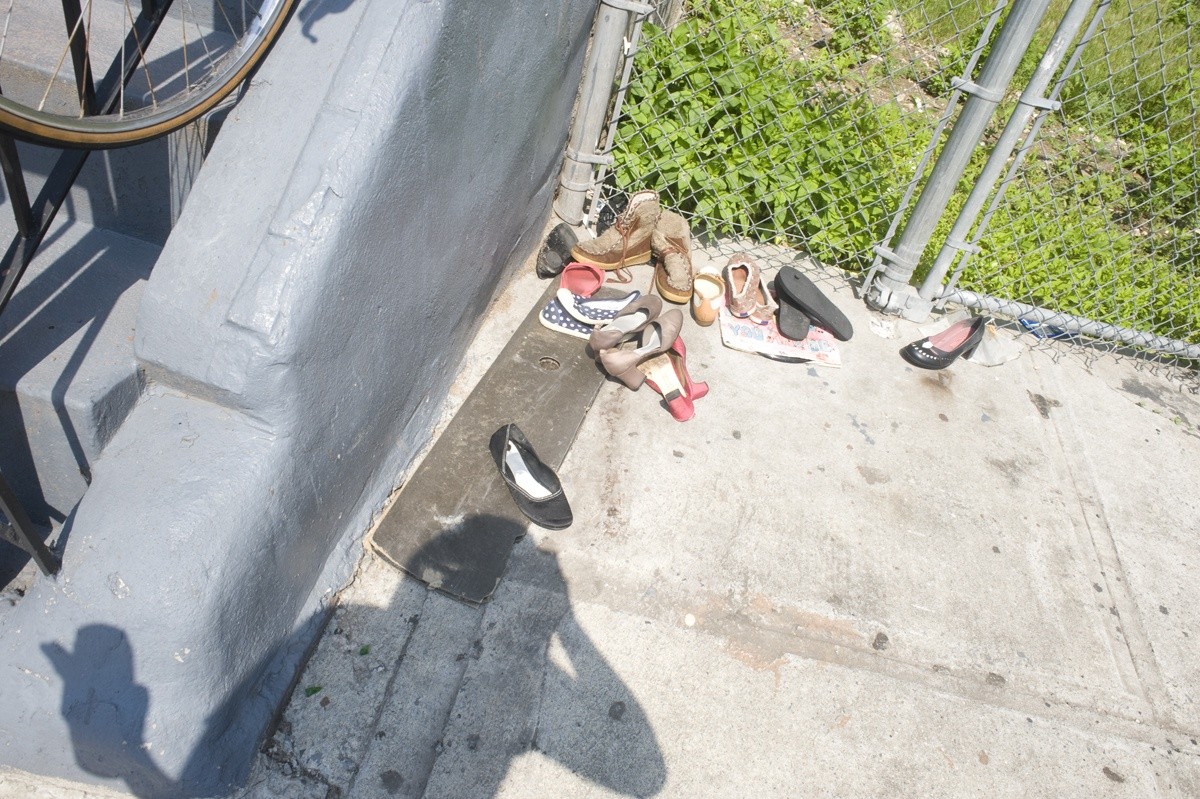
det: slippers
[559,263,605,297]
[775,277,811,341]
[774,267,853,341]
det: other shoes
[692,266,725,327]
[557,289,640,325]
[539,297,594,341]
[535,223,578,279]
[725,252,759,319]
[749,280,774,326]
[489,422,573,531]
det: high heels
[666,337,708,402]
[642,354,695,421]
[598,310,683,392]
[902,315,985,370]
[586,295,663,359]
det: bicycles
[0,0,307,151]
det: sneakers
[572,189,660,284]
[648,209,693,303]
